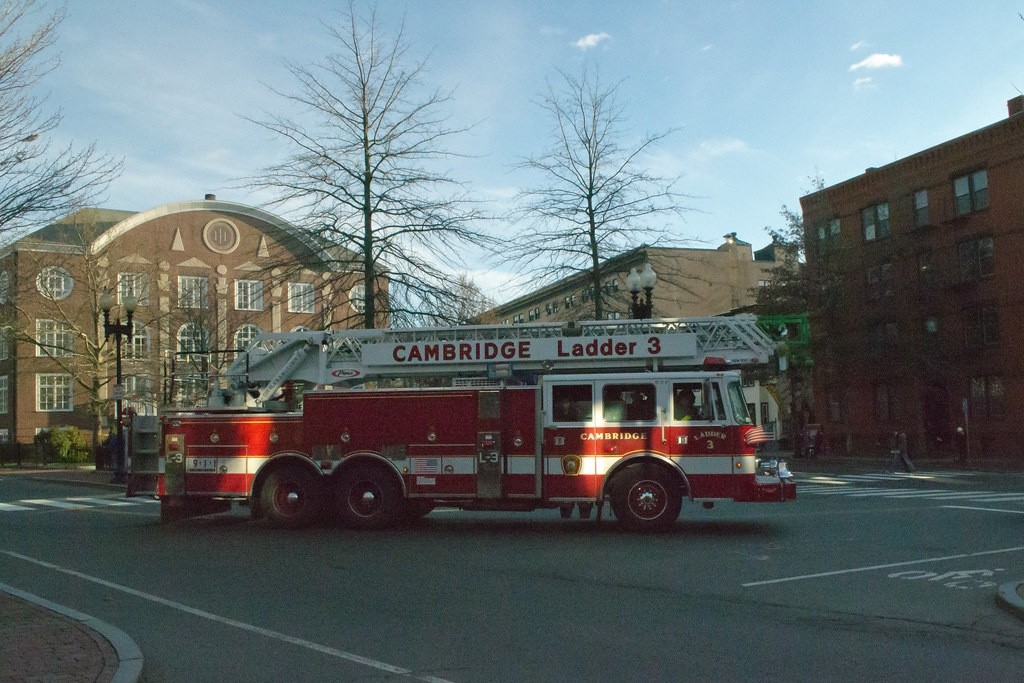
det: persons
[674,390,714,420]
[107,432,117,469]
[889,426,916,474]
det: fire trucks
[125,309,820,531]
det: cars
[97,434,128,469]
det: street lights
[100,286,138,483]
